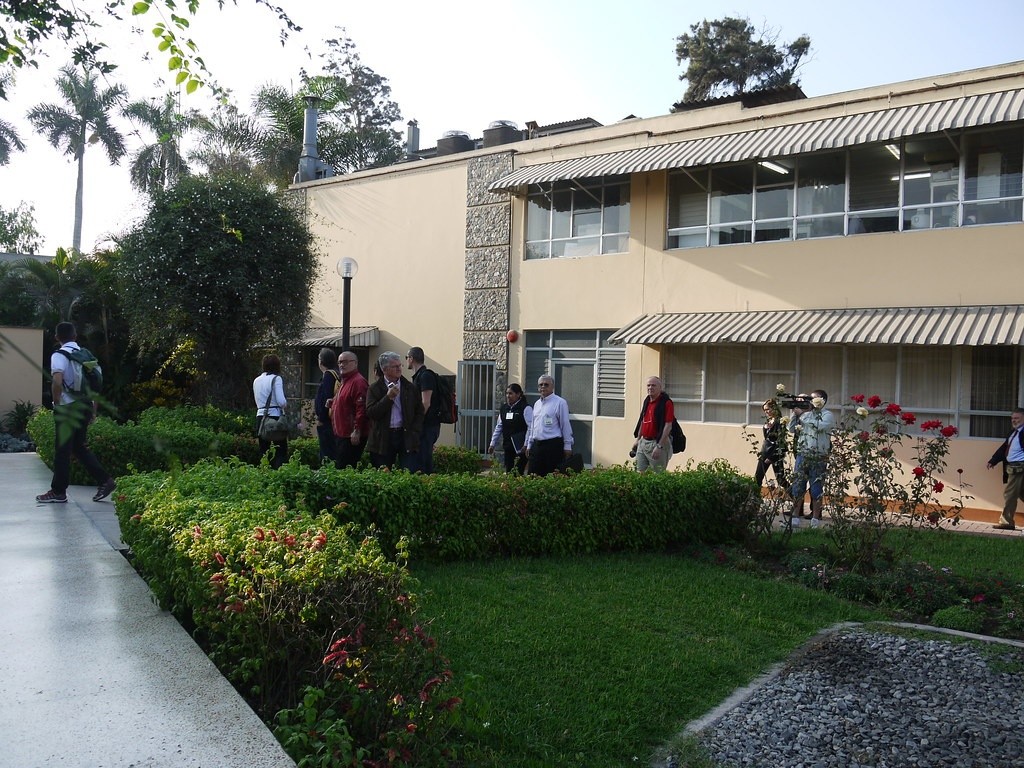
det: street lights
[337,257,359,352]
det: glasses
[538,383,553,388]
[405,356,411,360]
[337,359,356,366]
[384,365,405,370]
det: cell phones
[387,382,395,388]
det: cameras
[629,443,638,458]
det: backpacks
[322,369,341,416]
[55,347,103,401]
[416,369,458,424]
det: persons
[630,376,674,475]
[36,322,117,504]
[487,383,533,478]
[253,354,289,470]
[988,408,1024,530]
[525,374,574,477]
[751,389,834,530]
[315,347,440,475]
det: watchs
[656,444,663,449]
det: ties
[1005,430,1019,457]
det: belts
[1006,461,1024,466]
[644,437,658,441]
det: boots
[812,498,822,521]
[792,497,804,517]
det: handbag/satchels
[669,421,687,454]
[258,415,289,441]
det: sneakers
[93,478,118,501]
[37,491,68,503]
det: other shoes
[783,512,791,516]
[993,523,1016,530]
[805,511,813,519]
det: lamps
[885,144,902,161]
[757,161,790,175]
